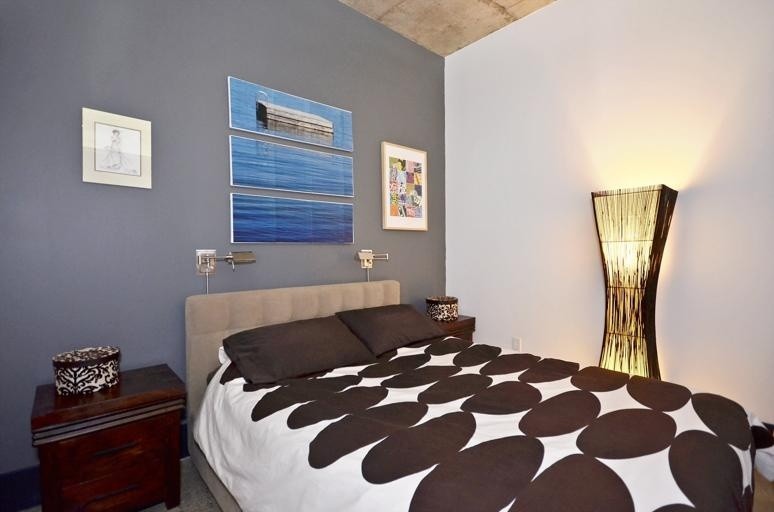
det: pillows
[222,302,444,388]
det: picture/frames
[78,105,153,191]
[379,138,430,234]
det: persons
[396,160,407,217]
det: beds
[183,278,755,512]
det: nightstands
[425,311,475,345]
[29,364,189,507]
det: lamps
[584,181,679,381]
